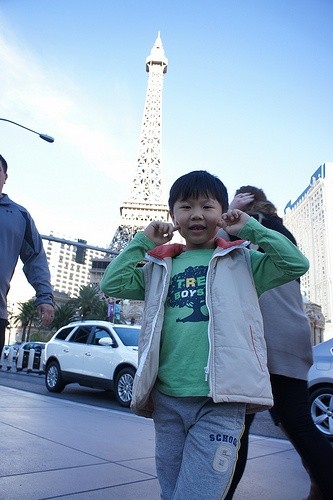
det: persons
[100,171,309,500]
[217,186,333,500]
[0,155,55,356]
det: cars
[16,342,45,371]
[4,342,23,361]
[307,337,333,441]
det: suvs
[38,319,142,407]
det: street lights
[0,117,54,143]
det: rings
[237,194,244,198]
[51,316,53,317]
